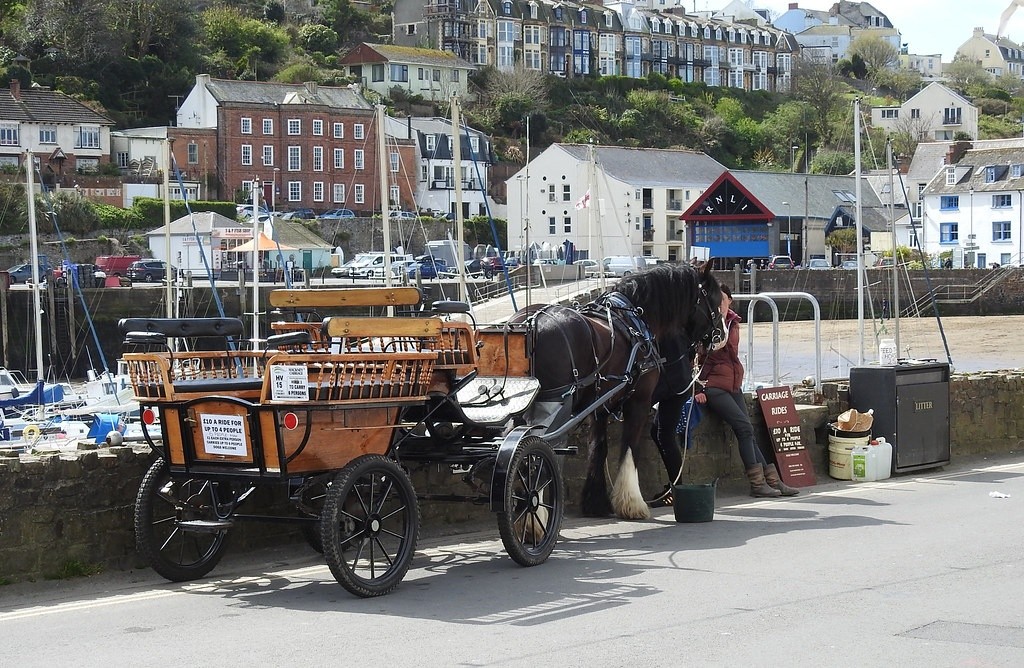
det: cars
[237,203,458,223]
[6,264,47,284]
[332,259,353,278]
[449,257,525,279]
[573,260,604,278]
[375,259,413,279]
[404,260,449,279]
[127,260,184,283]
[837,261,858,268]
[805,260,833,269]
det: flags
[575,184,591,210]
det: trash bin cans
[849,360,949,469]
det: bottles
[106,431,123,445]
[802,375,816,386]
[879,338,897,366]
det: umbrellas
[225,232,299,268]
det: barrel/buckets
[851,445,875,482]
[826,422,873,480]
[668,477,719,523]
[870,436,892,481]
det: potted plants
[825,228,856,253]
[390,84,405,101]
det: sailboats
[776,91,955,376]
[0,145,299,449]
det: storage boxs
[61,259,96,287]
[87,413,119,444]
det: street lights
[782,201,791,255]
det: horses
[504,255,731,523]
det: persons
[690,257,698,268]
[759,258,770,270]
[651,335,706,506]
[698,283,800,498]
[944,258,953,269]
[993,262,1000,270]
[259,255,262,263]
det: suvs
[873,259,895,266]
[770,255,794,270]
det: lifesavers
[22,425,40,441]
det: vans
[349,253,406,280]
[602,257,648,278]
[534,258,558,265]
[95,257,141,277]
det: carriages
[118,256,725,598]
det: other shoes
[650,484,674,507]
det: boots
[763,463,799,495]
[745,463,781,497]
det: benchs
[118,316,289,400]
[258,316,443,405]
[269,286,480,369]
